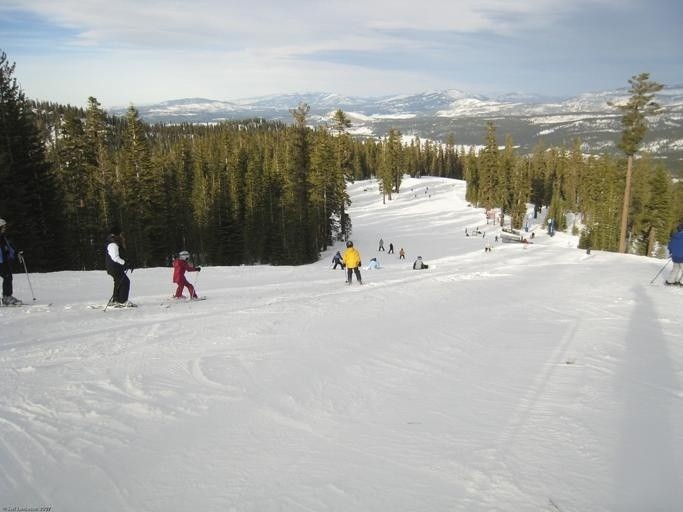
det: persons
[368,257,383,270]
[172,251,201,299]
[331,250,344,270]
[388,243,394,255]
[378,238,385,253]
[105,225,137,307]
[341,240,362,286]
[399,248,405,260]
[0,218,25,305]
[665,223,683,287]
[411,256,428,270]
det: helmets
[180,251,189,256]
[676,224,683,232]
[0,219,7,226]
[346,241,353,246]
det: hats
[110,226,121,234]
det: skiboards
[651,281,682,286]
[88,304,137,308]
[0,303,52,308]
[161,296,206,301]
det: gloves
[342,264,346,267]
[359,262,361,266]
[196,267,201,271]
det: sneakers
[2,296,21,305]
[113,300,134,306]
[359,281,362,284]
[191,295,198,299]
[346,281,350,283]
[176,295,186,299]
